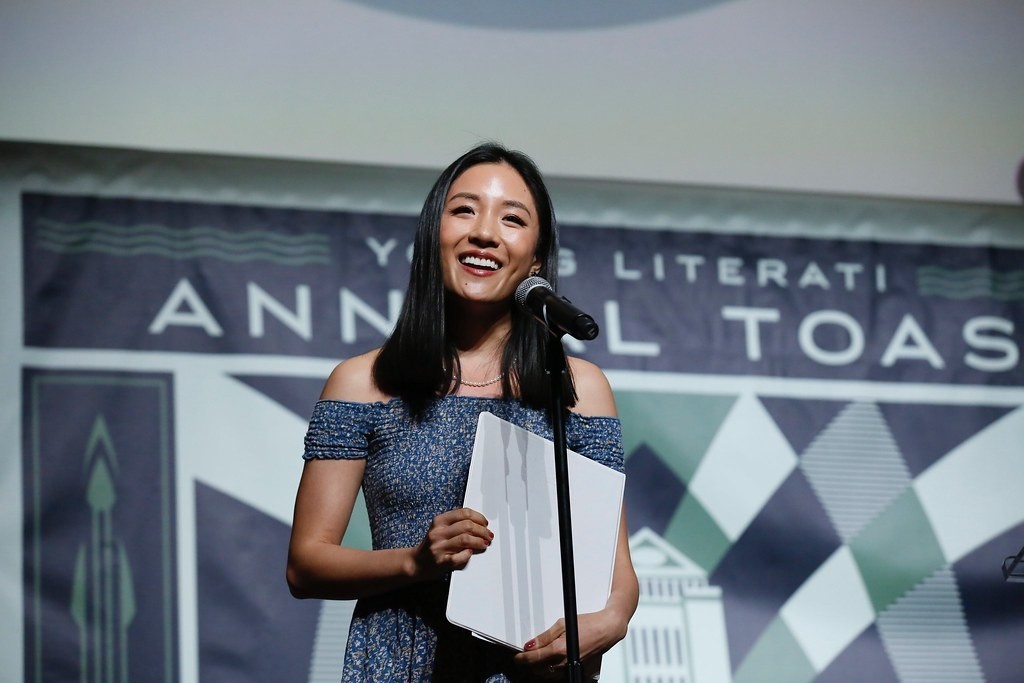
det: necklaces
[443,359,517,386]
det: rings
[547,664,555,673]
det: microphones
[516,276,599,341]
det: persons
[284,141,641,682]
